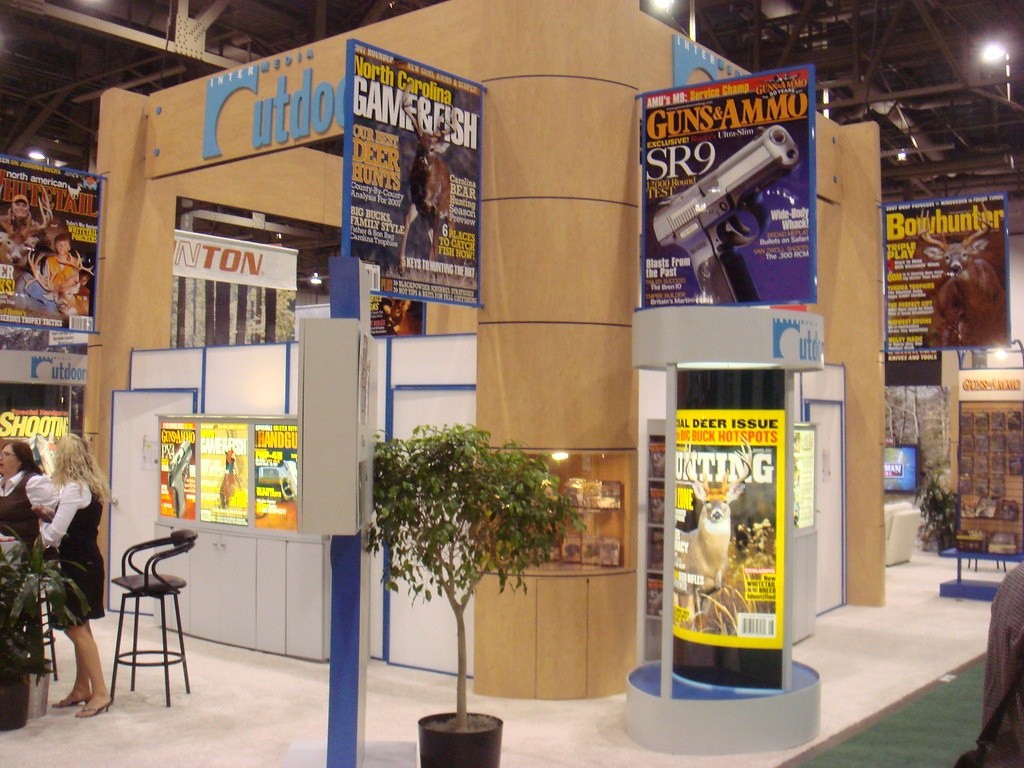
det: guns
[258,460,298,501]
[653,124,800,304]
[169,440,193,518]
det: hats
[12,194,28,204]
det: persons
[0,194,89,316]
[32,433,112,717]
[0,440,58,659]
[980,559,1024,768]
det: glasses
[1,451,16,457]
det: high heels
[75,697,111,718]
[51,693,91,708]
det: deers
[675,434,753,630]
[0,183,54,281]
[397,82,457,276]
[379,298,423,336]
[916,202,1006,347]
[19,246,96,318]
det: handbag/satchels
[954,751,983,768]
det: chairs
[882,500,921,567]
[110,529,200,709]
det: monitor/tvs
[884,443,923,494]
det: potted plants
[357,419,588,768]
[913,459,961,554]
[0,524,92,732]
